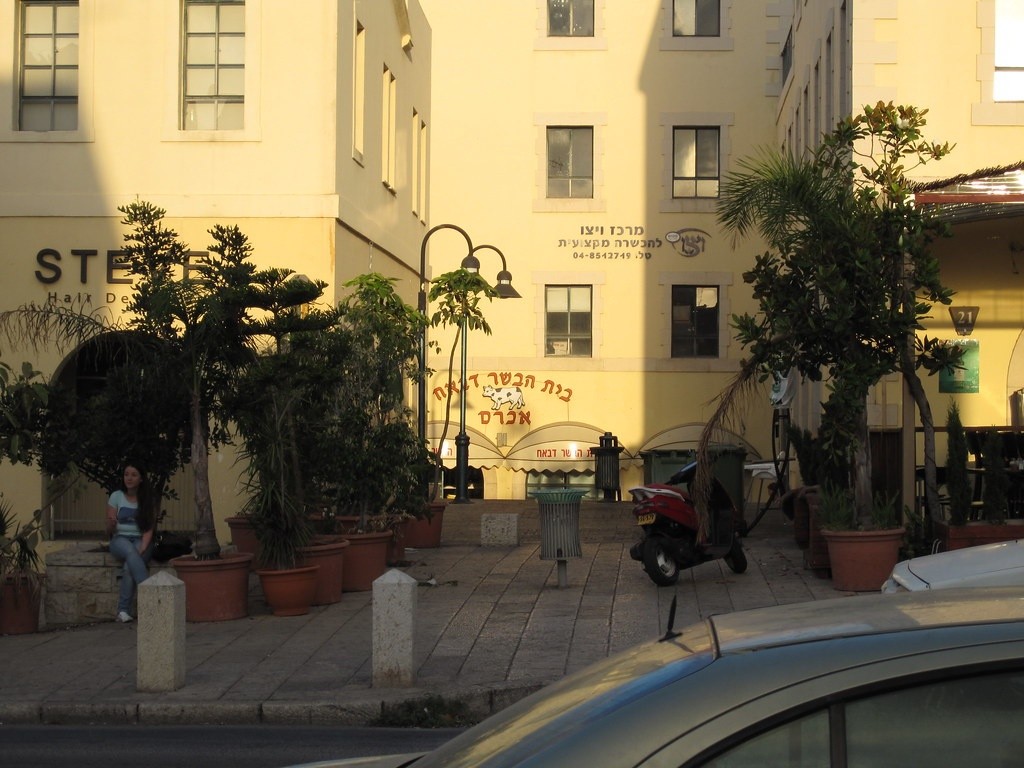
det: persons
[107,460,157,623]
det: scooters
[625,450,747,584]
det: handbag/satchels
[154,531,193,562]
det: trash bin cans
[528,488,589,561]
[637,443,747,523]
[589,433,625,502]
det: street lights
[416,223,484,515]
[454,244,520,505]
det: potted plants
[1,490,50,636]
[937,395,1024,551]
[119,200,500,621]
[783,416,904,591]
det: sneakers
[116,612,133,622]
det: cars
[879,538,1024,596]
[273,593,1024,768]
